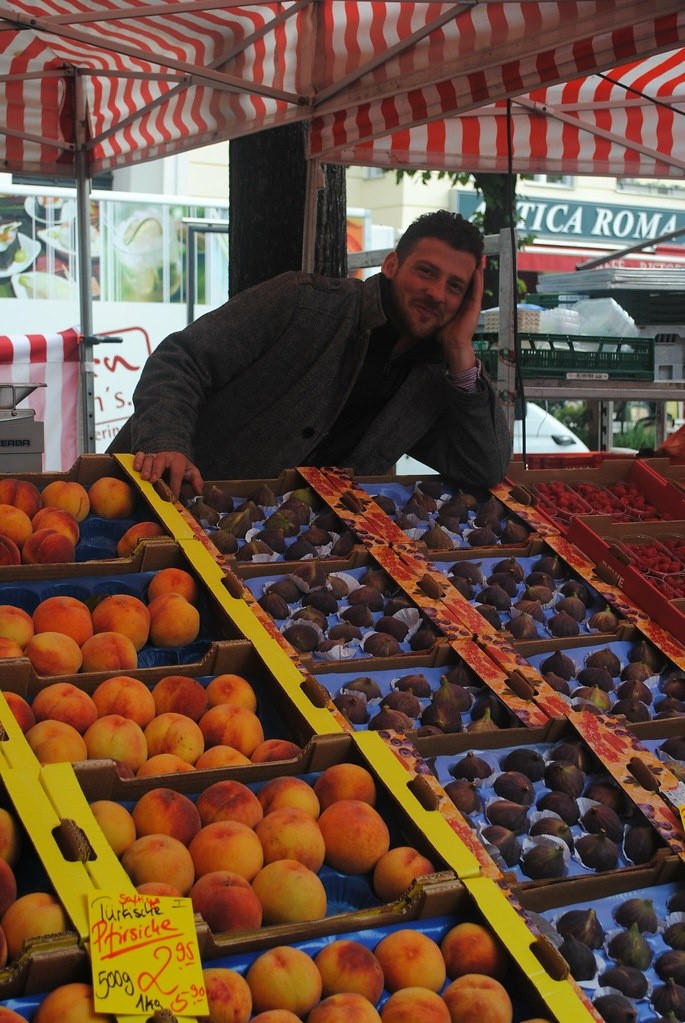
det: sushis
[0,195,77,301]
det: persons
[105,210,511,502]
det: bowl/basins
[0,383,47,409]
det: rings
[146,453,156,458]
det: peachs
[0,476,555,1023]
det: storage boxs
[472,332,656,384]
[0,453,684,1023]
